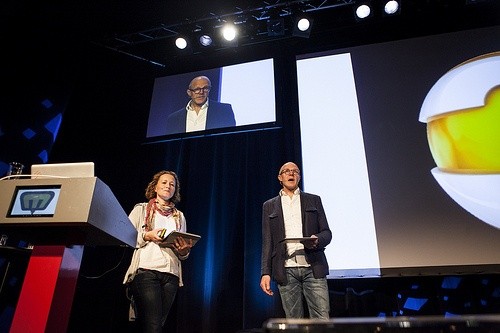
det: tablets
[279,237,318,244]
[162,230,201,248]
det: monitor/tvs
[143,56,283,144]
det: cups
[6,162,24,179]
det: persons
[260,162,333,329]
[122,169,202,330]
[165,75,237,133]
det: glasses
[280,169,300,175]
[189,87,209,94]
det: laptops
[30,162,94,179]
